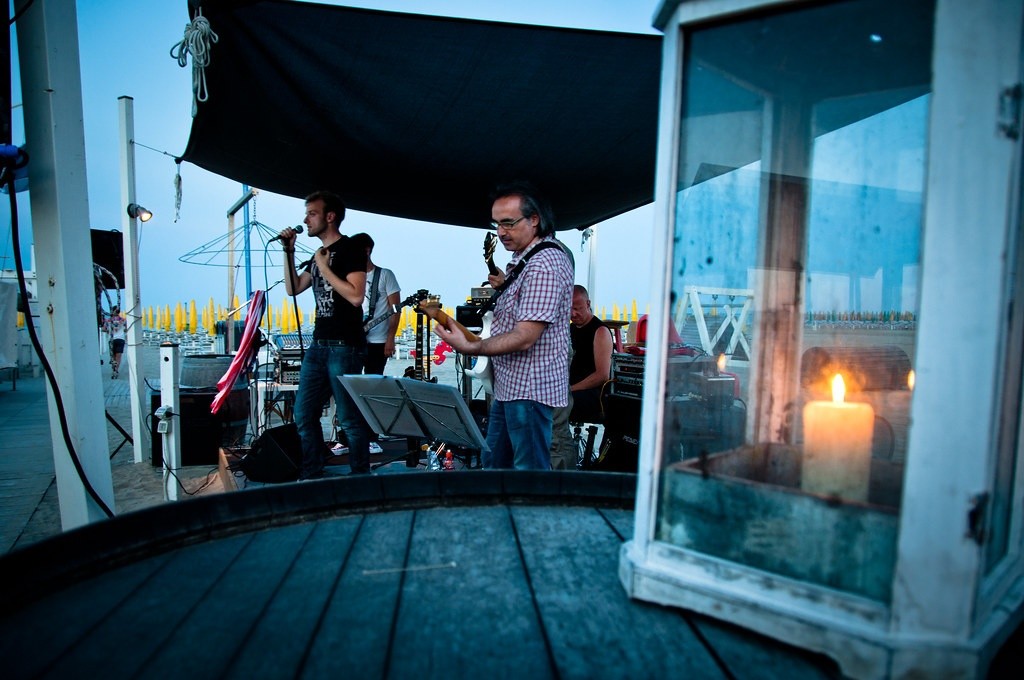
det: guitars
[482,231,499,276]
[419,293,494,395]
[364,289,431,336]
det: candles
[801,373,875,503]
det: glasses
[490,214,530,230]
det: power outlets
[155,406,173,420]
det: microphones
[268,225,303,242]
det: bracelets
[283,246,296,253]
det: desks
[250,379,336,440]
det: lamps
[127,204,152,222]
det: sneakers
[369,441,382,455]
[331,442,348,456]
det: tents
[177,2,934,222]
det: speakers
[149,389,250,468]
[90,228,125,290]
[240,421,335,484]
[597,389,734,476]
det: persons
[434,191,575,472]
[281,190,401,479]
[102,305,127,379]
[569,286,613,472]
